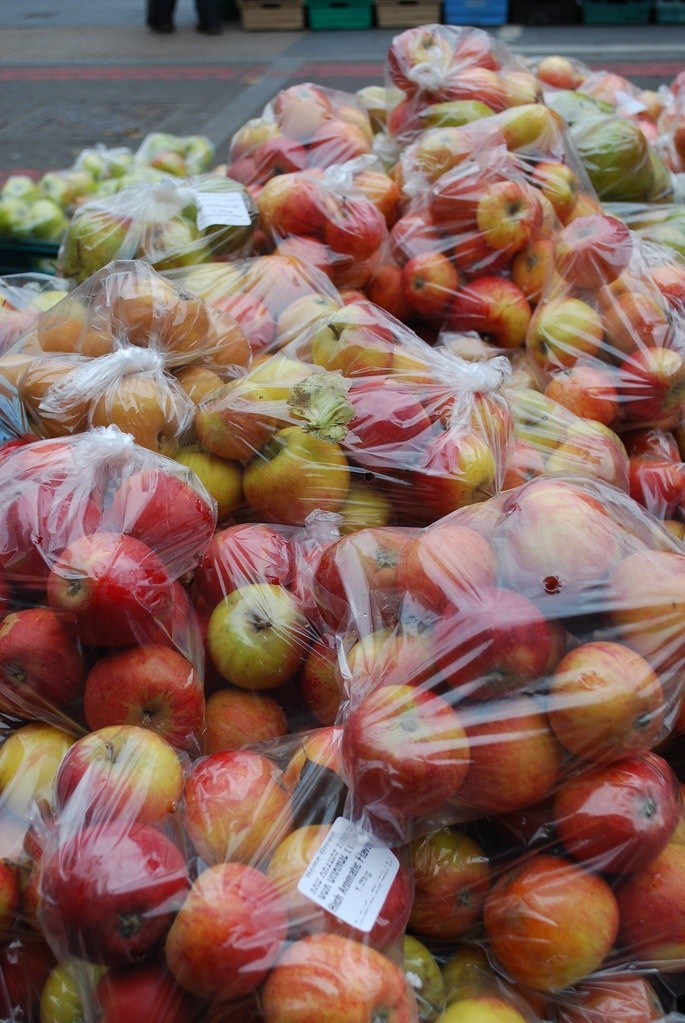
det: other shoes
[197,22,223,35]
[145,17,173,31]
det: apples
[0,23,685,1023]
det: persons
[148,0,223,34]
